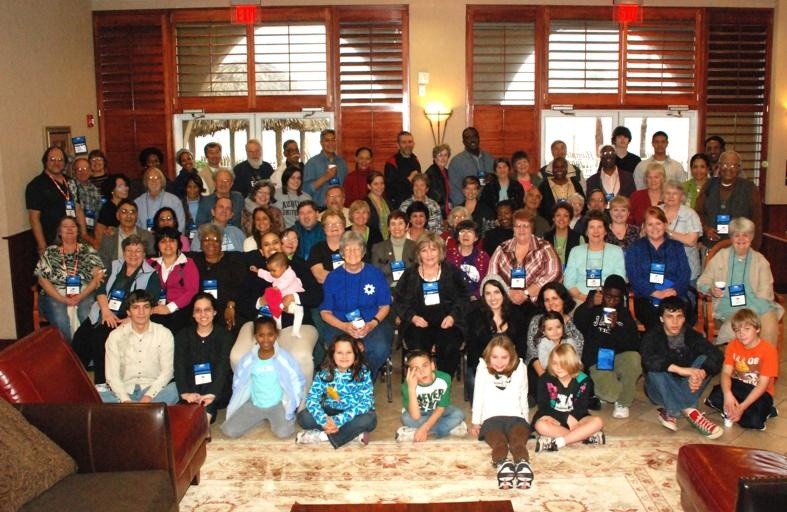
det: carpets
[174,436,705,512]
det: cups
[352,320,365,339]
[328,165,336,170]
[602,308,615,323]
[715,281,726,297]
[722,412,733,427]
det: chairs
[28,278,52,335]
[395,322,472,402]
[625,280,708,339]
[699,237,787,348]
[323,331,392,405]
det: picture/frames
[41,124,73,181]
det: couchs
[678,441,787,512]
[0,325,212,502]
[0,400,181,512]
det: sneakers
[295,428,322,444]
[395,426,417,442]
[514,458,533,489]
[358,431,370,445]
[658,409,678,432]
[535,434,559,453]
[496,456,516,489]
[612,401,630,419]
[687,408,724,440]
[582,429,605,445]
[449,421,468,439]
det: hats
[479,274,509,297]
[176,149,194,167]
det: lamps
[418,92,454,147]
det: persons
[470,336,535,489]
[26,125,780,453]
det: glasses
[285,148,299,153]
[115,176,230,241]
[723,163,741,169]
[283,193,344,243]
[453,215,531,234]
[48,157,104,171]
[569,151,612,206]
